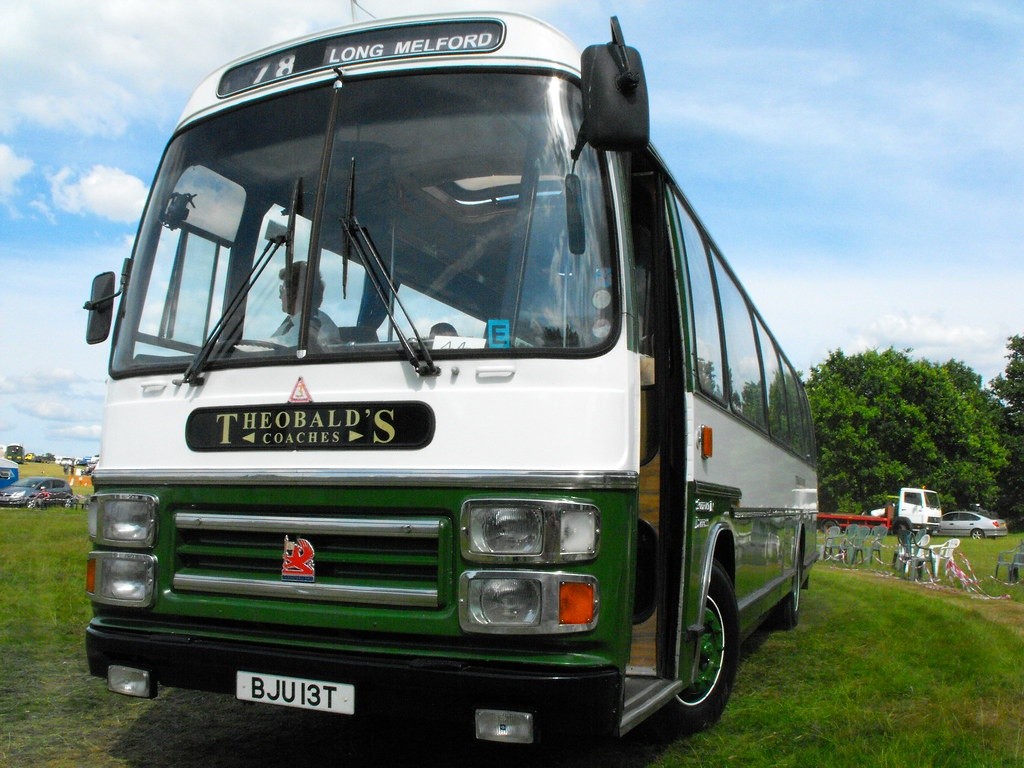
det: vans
[0,477,73,507]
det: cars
[934,509,1007,540]
[74,458,86,465]
[61,458,74,467]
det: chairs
[819,523,1024,584]
[336,324,380,344]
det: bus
[83,11,821,750]
[5,444,24,464]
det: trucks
[818,488,942,536]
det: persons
[271,260,340,344]
[428,322,459,339]
[34,486,49,511]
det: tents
[0,458,18,490]
